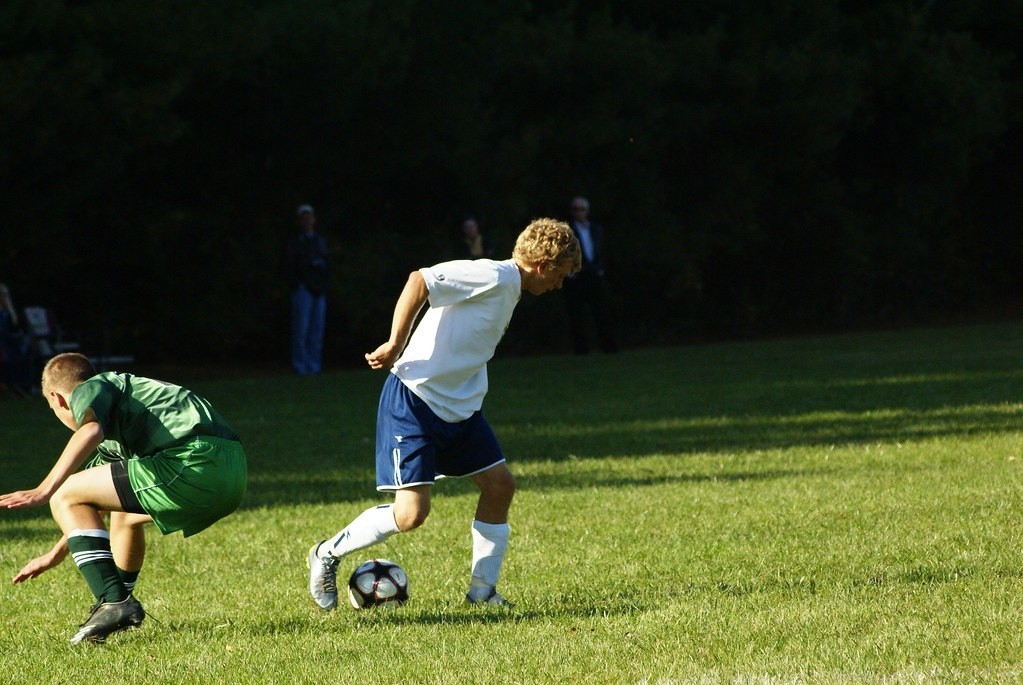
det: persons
[309,218,582,611]
[0,352,248,646]
[456,221,498,259]
[283,203,329,378]
[564,195,623,354]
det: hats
[297,204,314,216]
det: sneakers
[307,539,341,611]
[462,588,515,609]
[70,594,146,644]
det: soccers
[346,557,411,611]
